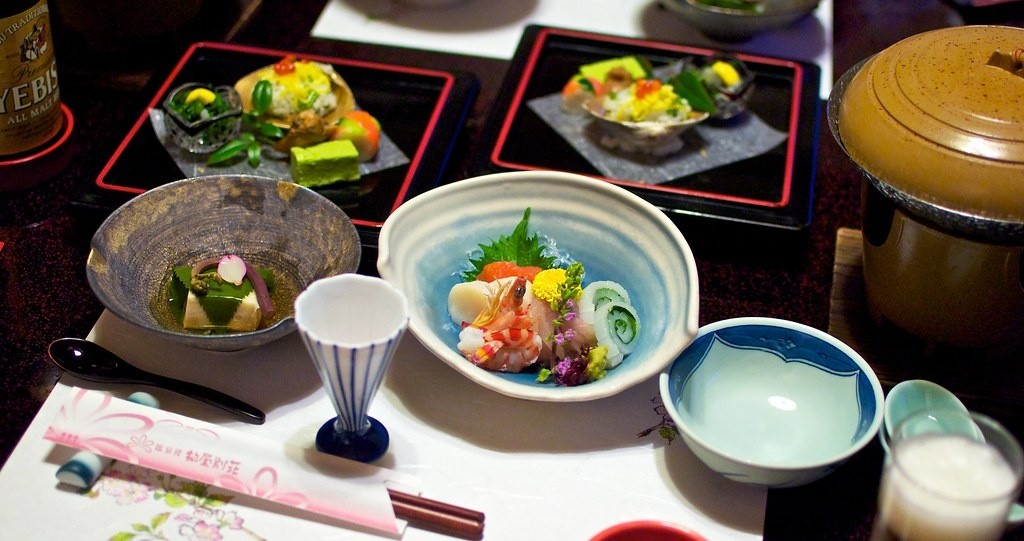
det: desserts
[230,61,380,189]
[559,56,693,127]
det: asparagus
[190,257,275,321]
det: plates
[884,378,975,446]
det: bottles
[1,1,61,159]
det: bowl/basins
[376,170,701,402]
[824,24,1024,246]
[659,1,820,33]
[86,174,361,355]
[582,94,708,147]
[660,316,885,484]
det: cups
[873,410,1024,541]
[162,82,243,154]
[682,52,756,120]
[293,272,411,463]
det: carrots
[477,261,544,284]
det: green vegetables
[170,80,318,168]
[461,205,563,284]
[661,56,745,121]
[171,267,273,333]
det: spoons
[46,338,266,424]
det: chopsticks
[387,486,487,540]
